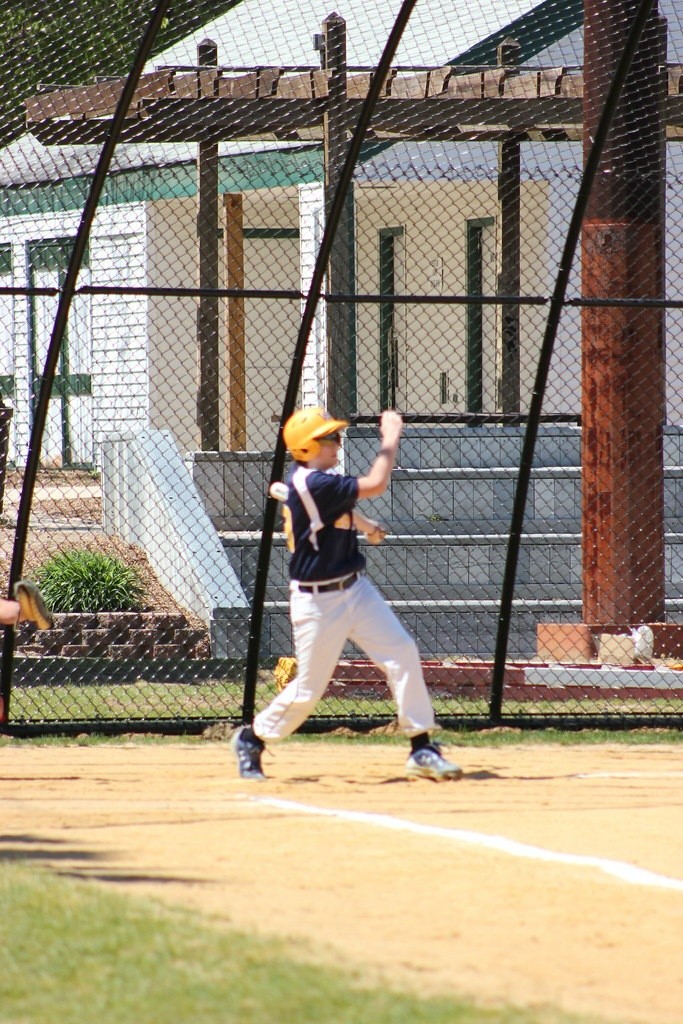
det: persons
[0,579,54,630]
[234,408,462,781]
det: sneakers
[410,743,463,776]
[231,727,265,779]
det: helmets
[283,408,347,462]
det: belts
[298,572,363,594]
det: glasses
[316,433,341,444]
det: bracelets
[367,526,377,537]
